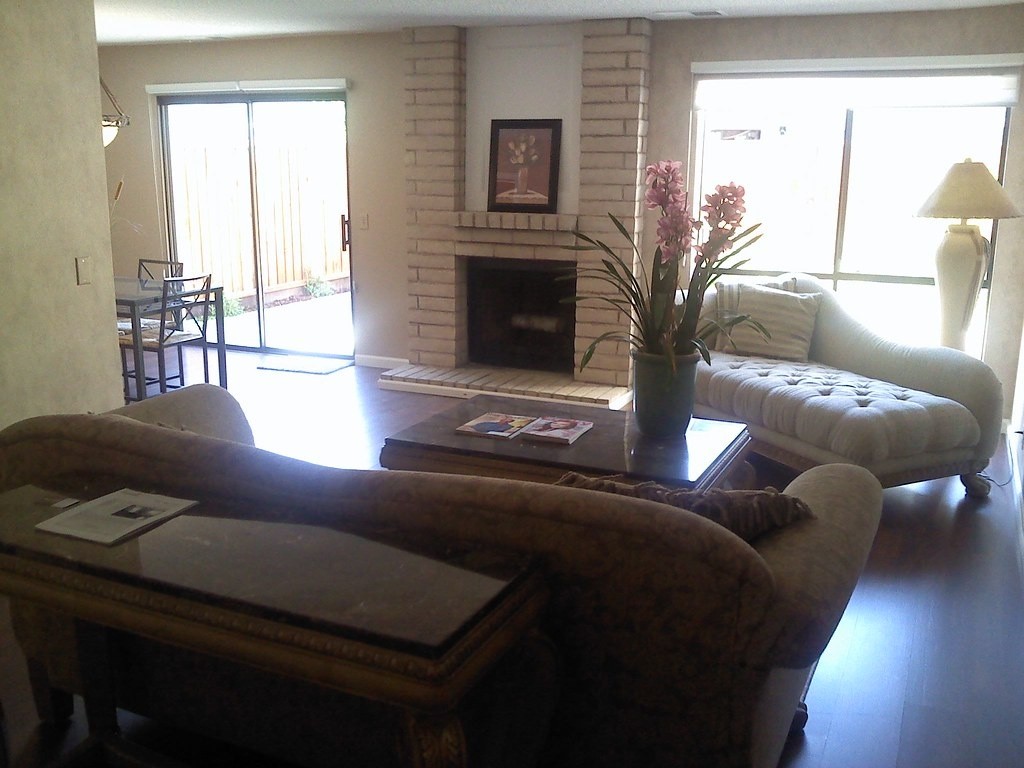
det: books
[456,412,542,440]
[520,415,594,445]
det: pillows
[722,284,822,364]
[714,277,798,354]
[554,471,817,541]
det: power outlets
[1016,428,1024,451]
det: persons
[472,416,515,432]
[533,420,577,438]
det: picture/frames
[486,119,563,214]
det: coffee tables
[378,392,758,540]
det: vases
[628,348,702,440]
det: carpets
[255,353,355,375]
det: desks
[114,275,228,400]
[0,480,552,768]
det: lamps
[98,76,129,147]
[916,158,1024,350]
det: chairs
[118,261,184,332]
[118,275,213,400]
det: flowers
[550,160,773,376]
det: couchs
[0,384,888,768]
[640,273,1004,500]
